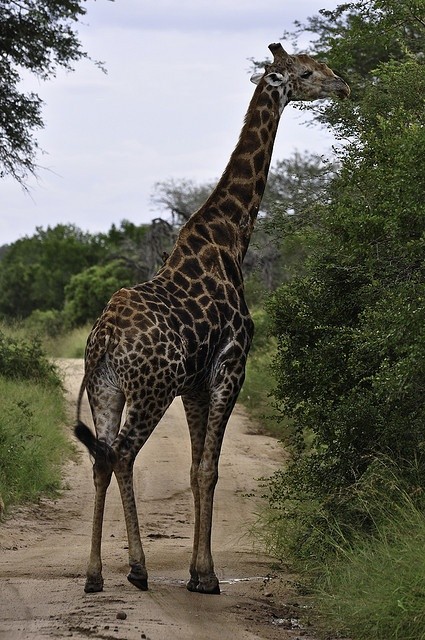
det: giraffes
[74,42,353,596]
